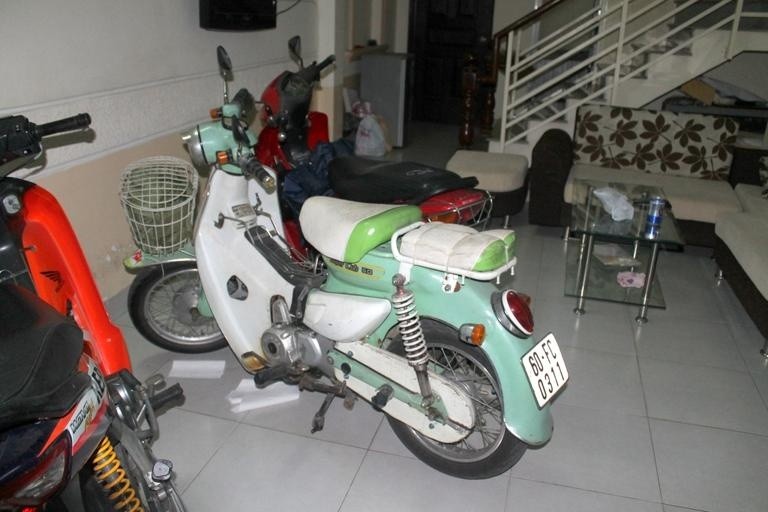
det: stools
[446,148,532,229]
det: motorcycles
[256,36,496,269]
[119,43,570,481]
[1,114,194,512]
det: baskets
[117,154,199,258]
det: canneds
[646,194,666,228]
[644,227,659,240]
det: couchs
[526,103,768,361]
